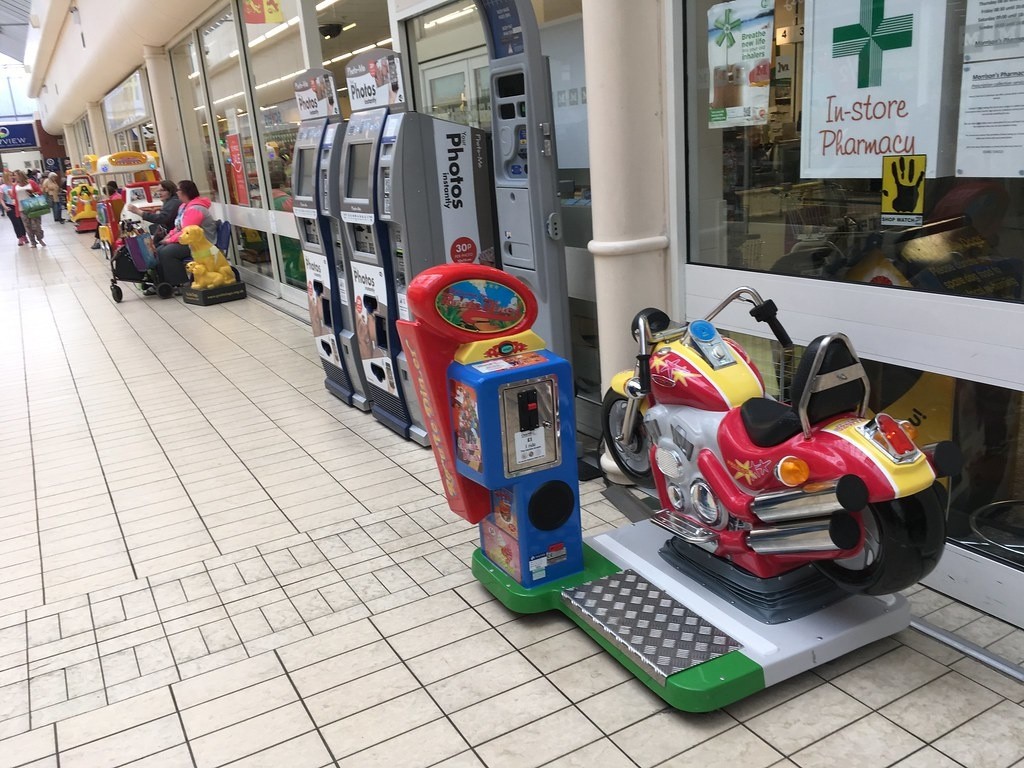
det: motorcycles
[601,287,946,596]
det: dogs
[177,225,237,289]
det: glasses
[156,190,167,192]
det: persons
[0,171,38,216]
[40,170,67,222]
[0,171,29,245]
[127,179,183,296]
[91,181,123,249]
[13,170,46,248]
[269,171,302,281]
[144,180,219,295]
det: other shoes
[143,284,158,295]
[18,236,24,246]
[39,240,46,247]
[91,243,101,249]
[22,234,28,243]
[54,218,66,221]
[2,214,5,216]
[31,241,36,247]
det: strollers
[98,218,172,302]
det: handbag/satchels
[21,191,51,219]
[123,220,161,272]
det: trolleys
[772,187,880,241]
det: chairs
[182,219,231,286]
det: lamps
[68,6,80,24]
[42,85,48,94]
